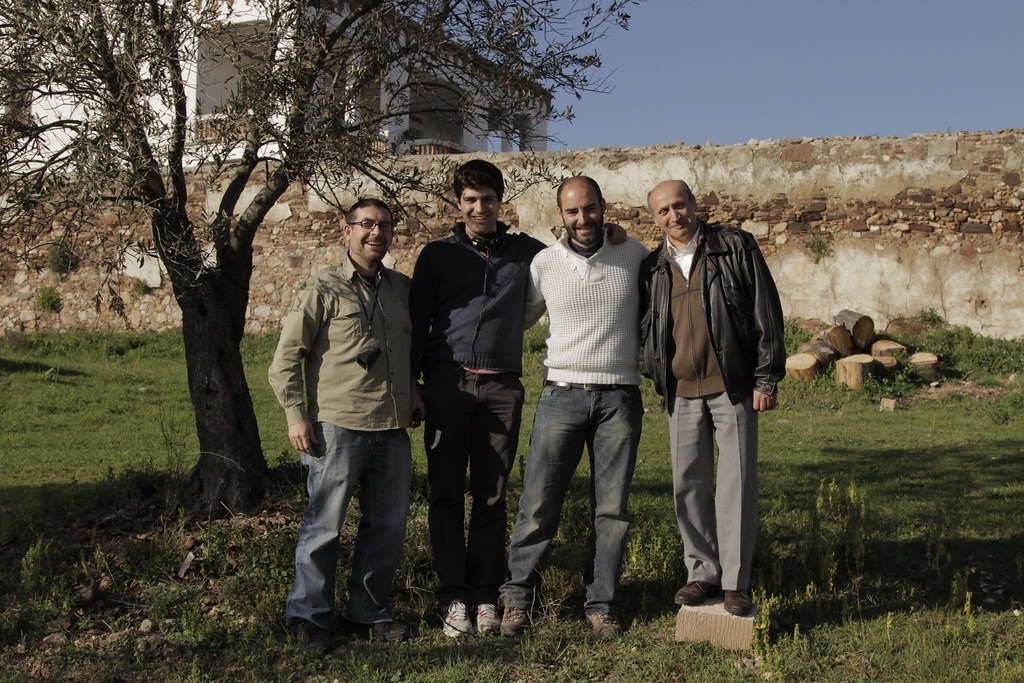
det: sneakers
[586,613,621,641]
[476,604,500,634]
[501,606,528,637]
[442,599,474,639]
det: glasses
[349,219,394,231]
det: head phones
[458,216,507,249]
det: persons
[638,179,787,616]
[500,175,653,637]
[409,159,549,637]
[267,199,411,643]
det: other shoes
[724,588,752,615]
[345,616,408,642]
[295,623,332,652]
[675,581,720,606]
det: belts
[545,382,626,392]
